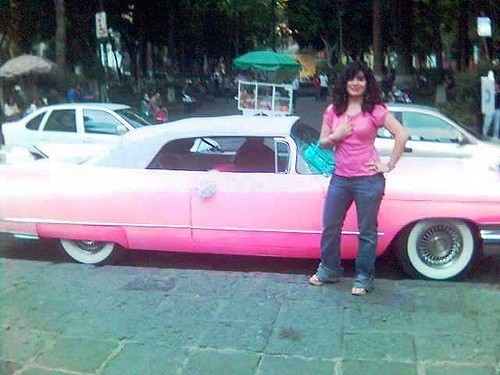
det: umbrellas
[0,55,51,79]
[233,50,300,73]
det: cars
[2,102,221,159]
[374,103,500,158]
[0,116,499,282]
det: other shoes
[309,274,327,285]
[351,287,366,295]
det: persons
[4,83,194,127]
[290,71,328,114]
[483,81,500,140]
[310,61,409,296]
